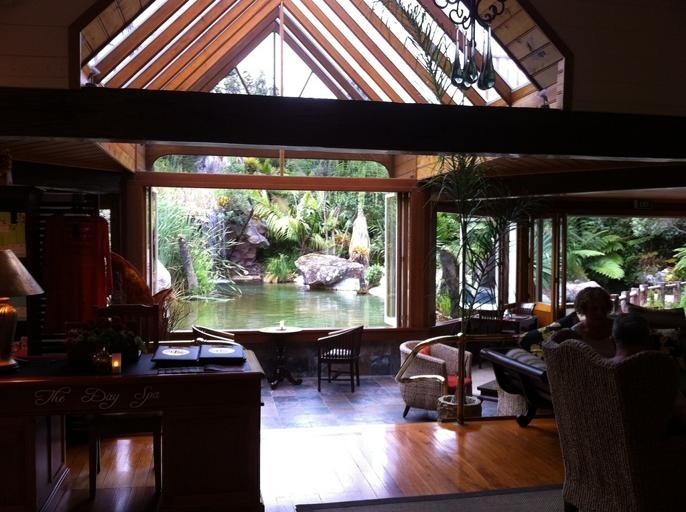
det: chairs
[88,303,161,500]
[399,340,472,419]
[543,327,685,512]
[481,347,555,418]
[316,324,364,393]
[477,309,504,367]
[517,303,536,315]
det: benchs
[191,325,235,341]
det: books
[151,345,246,366]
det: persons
[570,286,616,357]
[611,312,649,361]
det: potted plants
[354,0,539,423]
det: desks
[0,348,264,512]
[258,326,304,390]
[462,313,538,364]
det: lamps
[0,248,45,361]
[432,1,504,90]
